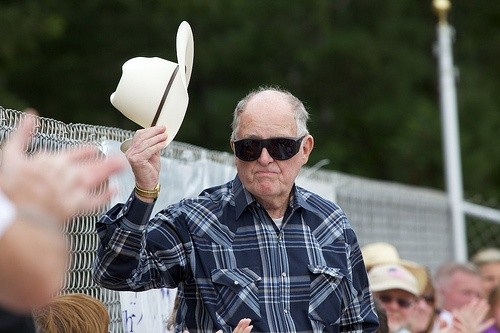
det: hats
[110,21,194,153]
[368,264,419,299]
[360,243,428,296]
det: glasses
[378,294,412,308]
[419,293,435,304]
[233,137,304,161]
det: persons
[0,87,500,333]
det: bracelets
[135,183,160,198]
